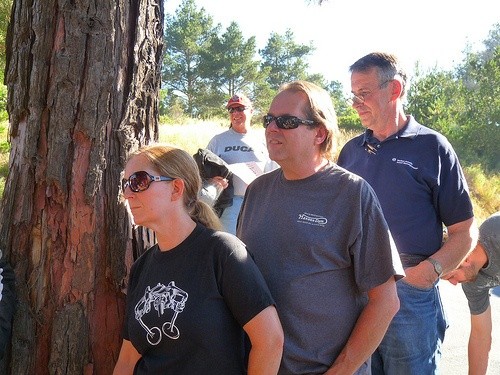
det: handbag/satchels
[193,149,233,217]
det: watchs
[426,257,443,278]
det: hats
[226,95,251,108]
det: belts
[400,255,428,267]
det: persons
[440,210,500,375]
[336,51,480,375]
[112,146,284,375]
[235,81,406,375]
[193,95,280,237]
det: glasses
[263,115,317,129]
[351,79,391,104]
[122,171,176,193]
[228,107,250,113]
